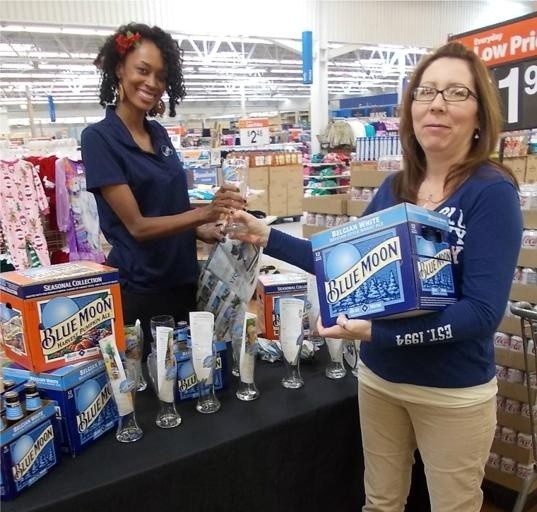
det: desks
[1,340,358,512]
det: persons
[80,20,247,363]
[222,41,522,512]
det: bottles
[227,149,302,167]
[0,374,43,434]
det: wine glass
[220,159,249,233]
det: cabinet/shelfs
[300,154,536,499]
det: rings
[342,319,348,330]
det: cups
[107,295,347,443]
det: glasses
[411,86,479,101]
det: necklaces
[421,178,446,207]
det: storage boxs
[308,201,460,331]
